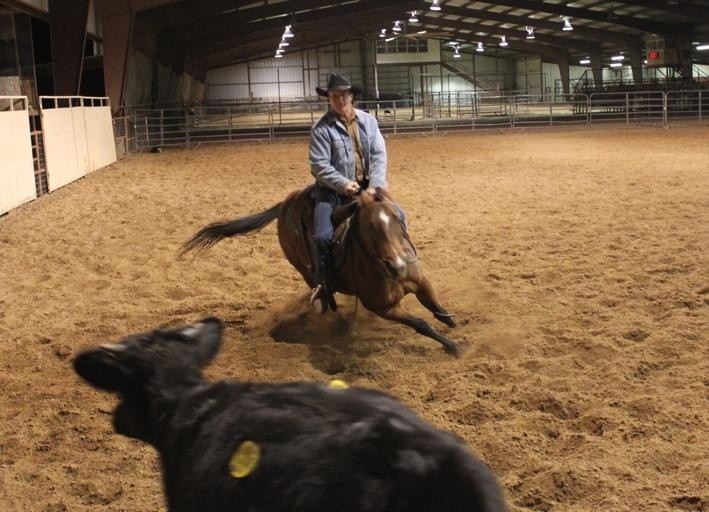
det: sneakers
[311,280,328,314]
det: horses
[171,181,461,359]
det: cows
[69,314,508,511]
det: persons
[407,88,414,107]
[309,72,405,313]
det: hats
[316,72,363,96]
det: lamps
[451,15,574,59]
[275,25,294,61]
[380,0,441,38]
